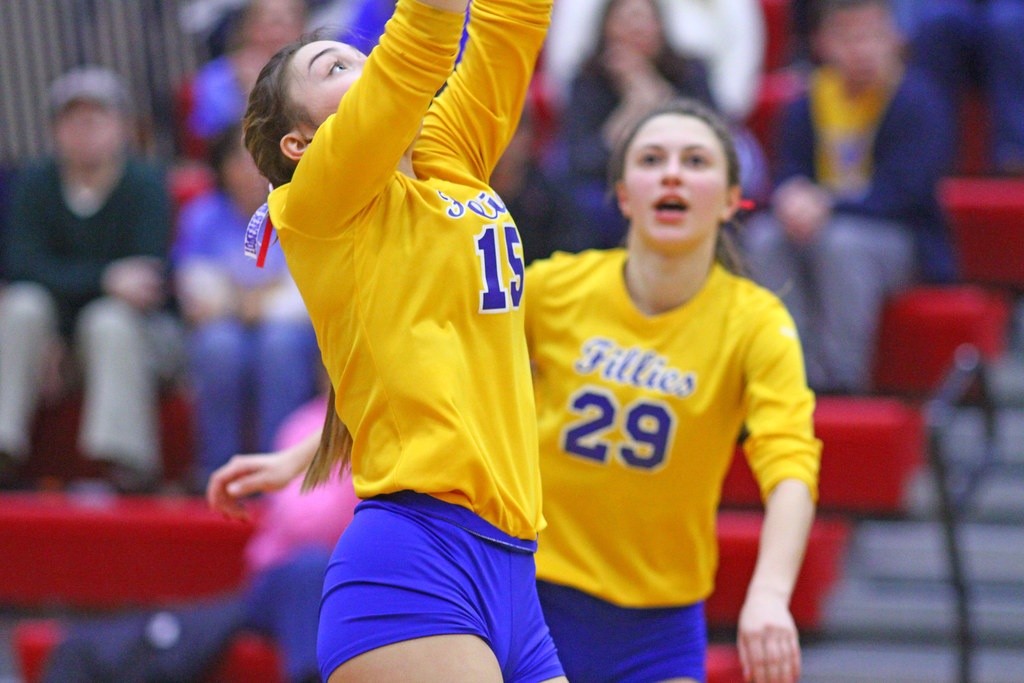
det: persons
[0,64,188,496]
[194,95,826,683]
[0,0,1024,683]
[236,0,574,683]
[729,0,958,399]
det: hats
[44,63,122,116]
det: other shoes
[0,450,34,491]
[78,461,163,500]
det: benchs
[0,0,1024,683]
